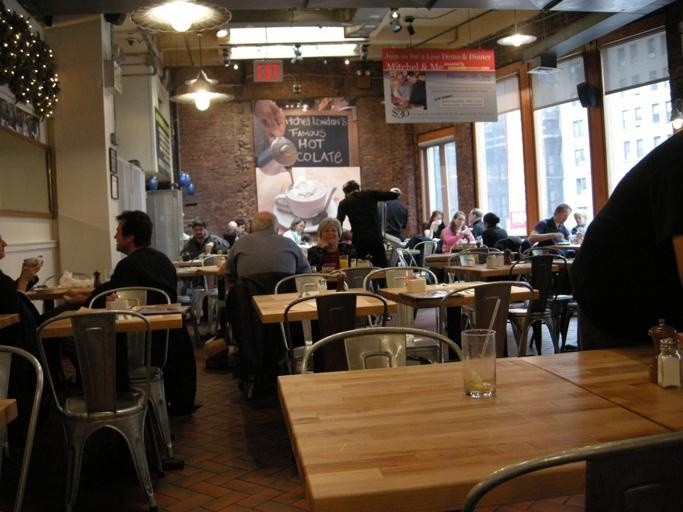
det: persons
[337,181,400,289]
[63,210,177,355]
[394,72,426,112]
[528,203,587,296]
[183,215,252,321]
[571,120,683,350]
[218,211,312,286]
[0,232,44,338]
[378,188,409,242]
[256,102,287,145]
[280,216,352,271]
[422,208,508,282]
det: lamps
[168,35,236,111]
[185,35,219,87]
[130,0,232,35]
[497,10,538,49]
[390,8,402,33]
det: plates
[32,287,53,293]
[271,199,339,231]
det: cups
[176,256,181,261]
[458,329,497,400]
[338,255,347,268]
[435,220,440,224]
[204,245,211,255]
[486,256,496,268]
[310,264,335,273]
[568,235,576,244]
[350,258,356,267]
[273,179,327,220]
[447,272,455,292]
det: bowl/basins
[24,255,43,268]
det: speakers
[577,82,598,107]
[104,12,127,25]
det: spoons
[311,188,336,226]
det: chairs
[462,430,683,511]
[297,330,469,375]
[196,254,236,333]
[511,255,574,354]
[270,271,351,291]
[447,246,503,278]
[432,280,533,358]
[363,264,437,287]
[396,240,439,263]
[30,313,162,508]
[282,289,388,329]
[88,284,175,469]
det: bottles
[646,320,674,383]
[335,271,348,290]
[477,236,482,248]
[318,279,327,295]
[93,270,100,288]
[503,249,512,265]
[217,249,222,255]
[105,295,129,318]
[656,337,682,391]
